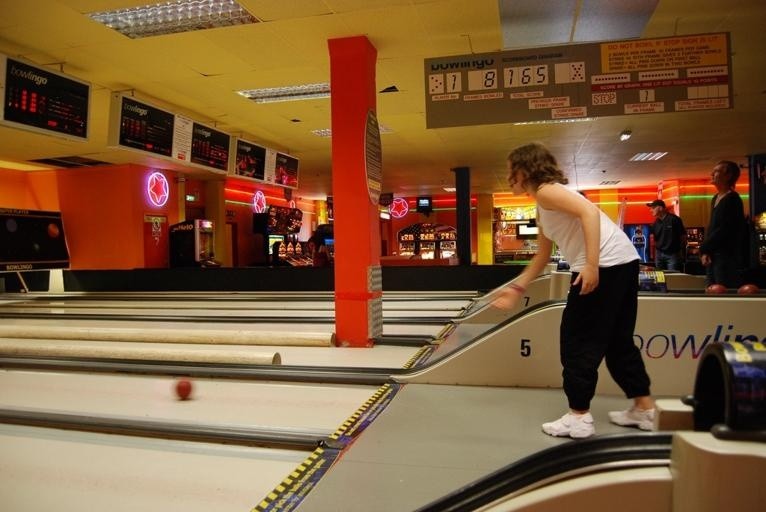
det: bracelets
[508,282,528,294]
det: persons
[646,199,689,274]
[488,141,664,439]
[308,229,331,267]
[697,160,748,289]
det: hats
[646,199,665,208]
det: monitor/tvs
[416,197,432,212]
[269,235,284,255]
[199,232,213,259]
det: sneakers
[607,406,657,431]
[542,410,596,439]
